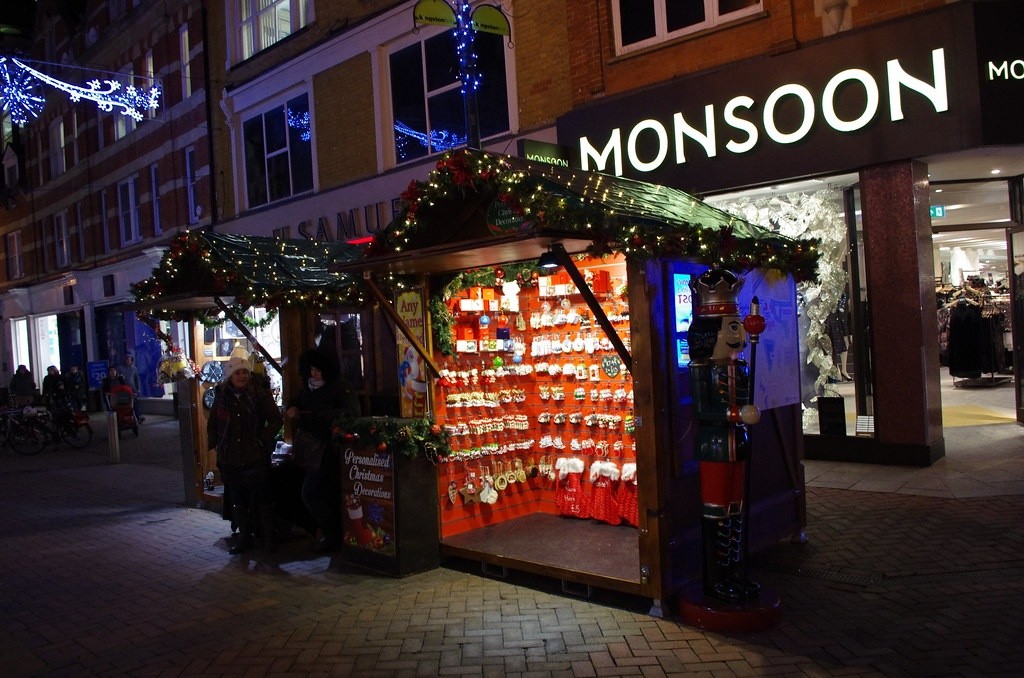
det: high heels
[841,370,853,381]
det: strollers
[105,386,140,440]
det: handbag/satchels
[251,404,283,442]
[284,428,327,473]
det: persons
[208,357,283,553]
[102,366,126,410]
[116,354,145,424]
[691,262,760,601]
[10,365,36,396]
[826,305,852,382]
[43,366,84,404]
[286,346,361,554]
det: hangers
[945,286,1000,318]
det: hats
[224,358,253,381]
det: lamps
[536,245,562,268]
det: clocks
[204,388,219,410]
[202,360,225,383]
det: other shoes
[228,528,254,554]
[308,535,343,554]
[263,526,281,554]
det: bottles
[207,470,215,491]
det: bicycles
[0,398,93,456]
